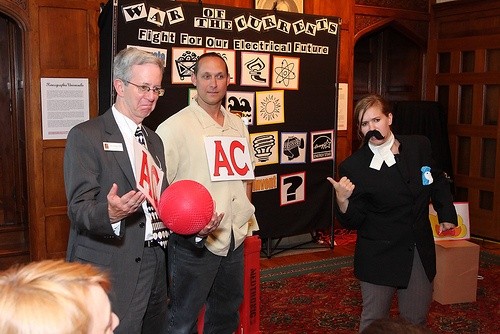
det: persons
[155,53,256,334]
[0,259,120,334]
[326,94,459,334]
[63,47,225,334]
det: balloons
[158,179,213,235]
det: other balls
[158,180,213,235]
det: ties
[134,127,171,251]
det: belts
[144,239,158,248]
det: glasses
[118,77,165,96]
